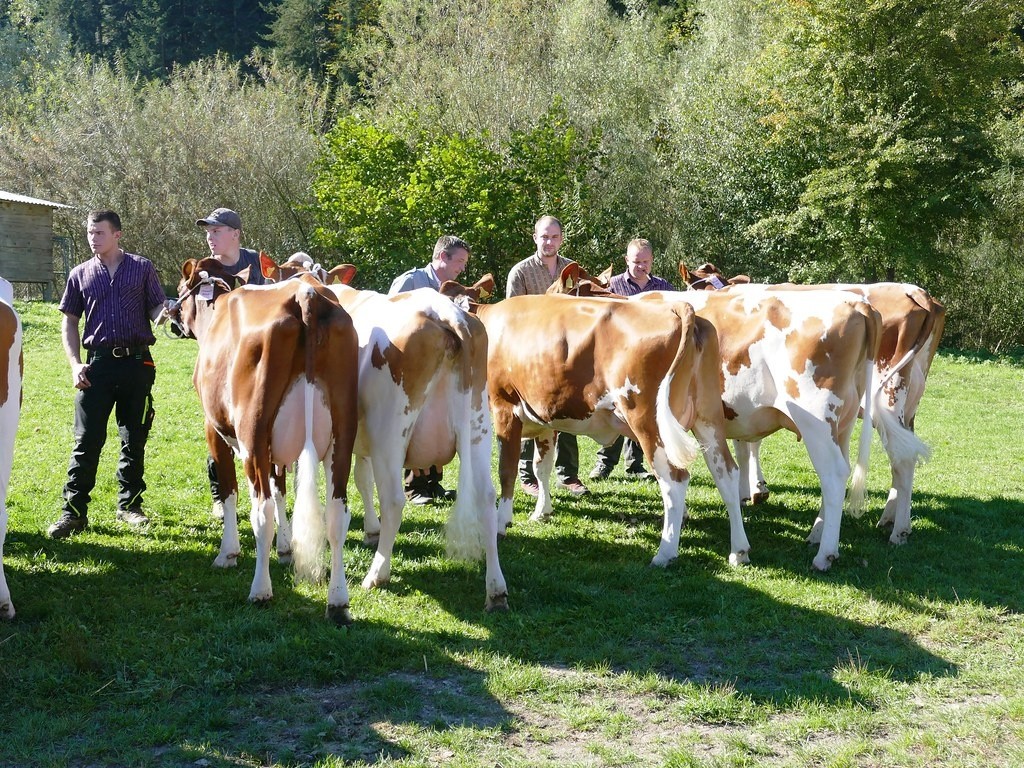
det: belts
[87,346,150,358]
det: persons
[175,208,268,523]
[501,216,592,503]
[284,249,314,277]
[383,234,470,508]
[593,236,671,481]
[49,210,184,540]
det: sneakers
[49,510,88,538]
[115,509,150,527]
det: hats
[196,207,241,229]
[279,252,313,267]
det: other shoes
[521,481,552,497]
[408,480,456,506]
[624,466,657,481]
[213,500,238,521]
[589,465,611,482]
[556,477,592,497]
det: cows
[172,257,359,626]
[0,276,25,621]
[439,273,753,571]
[258,248,511,615]
[543,260,884,574]
[678,261,946,549]
